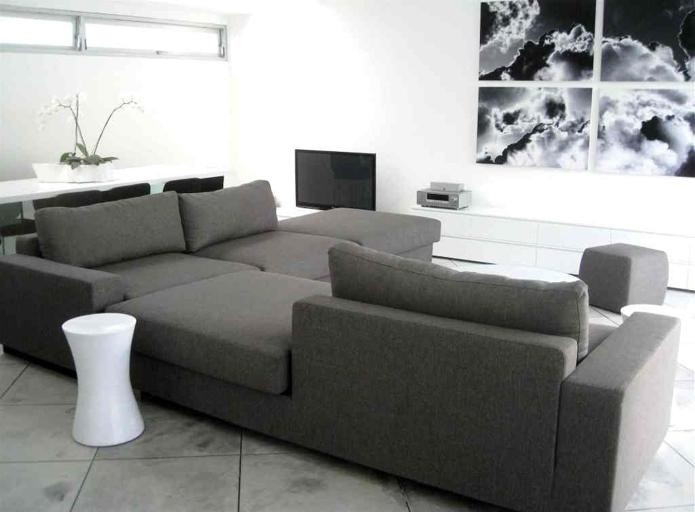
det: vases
[30,162,117,184]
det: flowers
[31,87,153,170]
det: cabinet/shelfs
[410,202,692,296]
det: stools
[575,240,670,316]
[61,312,146,449]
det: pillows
[325,238,591,361]
[33,191,187,268]
[176,179,279,254]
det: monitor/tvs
[294,149,377,211]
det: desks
[0,162,228,220]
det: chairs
[0,177,36,255]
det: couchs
[1,172,683,512]
